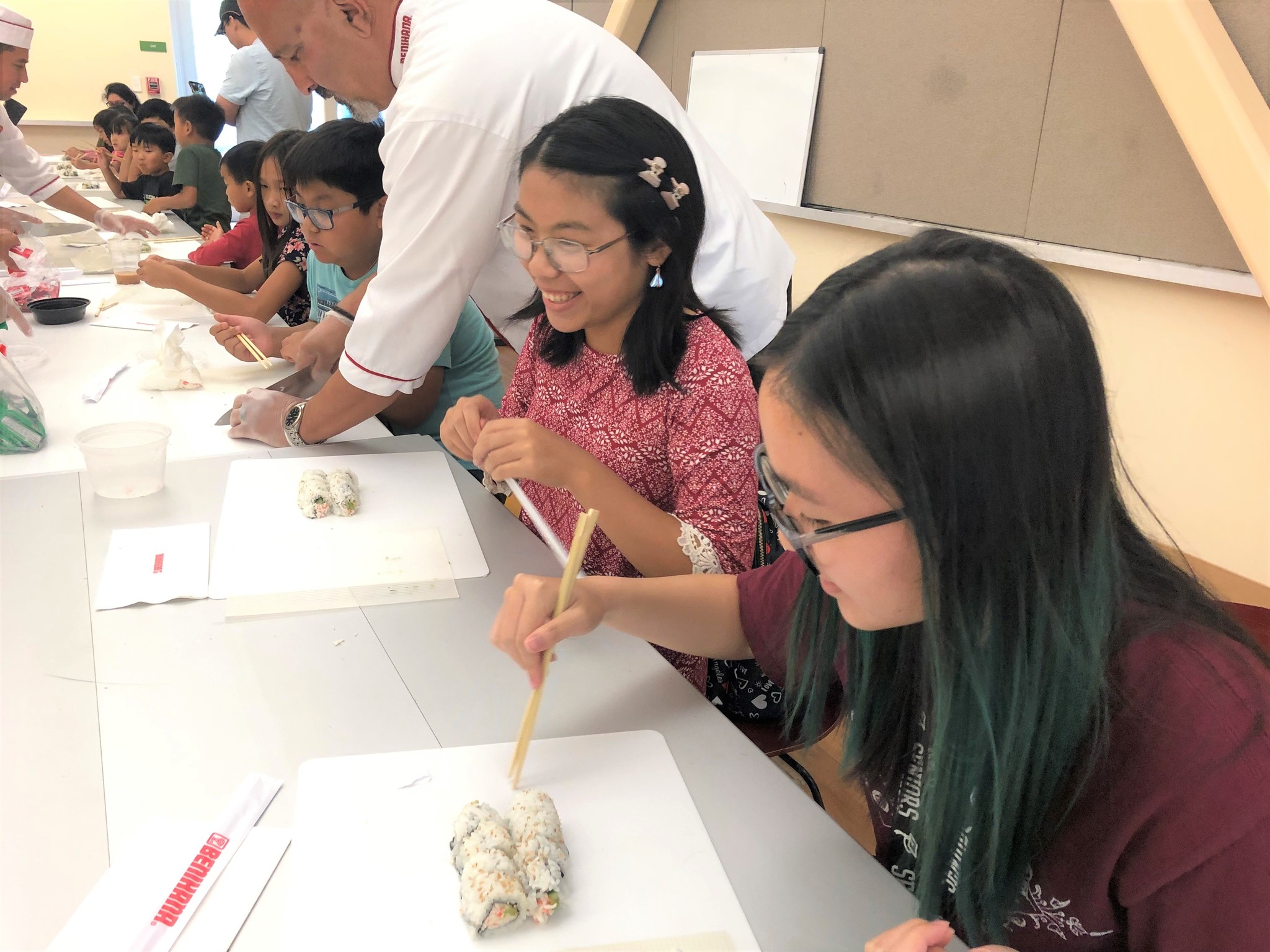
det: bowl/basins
[26,297,91,325]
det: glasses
[106,101,127,108]
[221,19,230,36]
[284,194,380,230]
[495,211,634,274]
[752,442,911,577]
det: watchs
[283,401,327,447]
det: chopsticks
[68,153,88,164]
[507,508,599,789]
[94,299,119,317]
[77,141,126,163]
[231,323,275,370]
[145,235,202,243]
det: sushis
[56,154,100,189]
[296,468,362,518]
[449,787,571,935]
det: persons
[237,0,795,447]
[438,96,763,699]
[491,229,1270,952]
[0,1,504,491]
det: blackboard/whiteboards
[0,0,178,127]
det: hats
[0,6,34,49]
[214,0,242,36]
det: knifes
[20,222,93,237]
[215,360,333,427]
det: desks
[0,151,965,951]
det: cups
[73,421,172,498]
[108,238,140,285]
